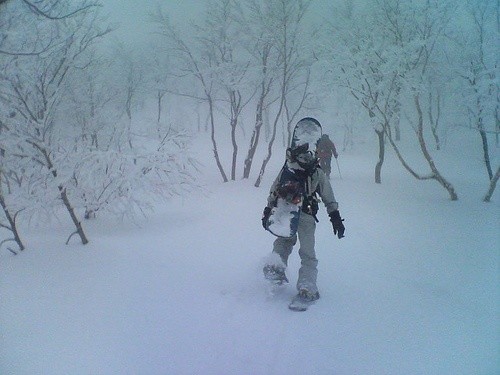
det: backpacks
[278,143,322,223]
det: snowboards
[265,117,322,238]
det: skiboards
[263,265,319,311]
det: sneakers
[267,263,289,282]
[298,289,319,302]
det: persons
[262,153,346,302]
[315,135,339,179]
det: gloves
[261,206,271,230]
[329,210,345,238]
